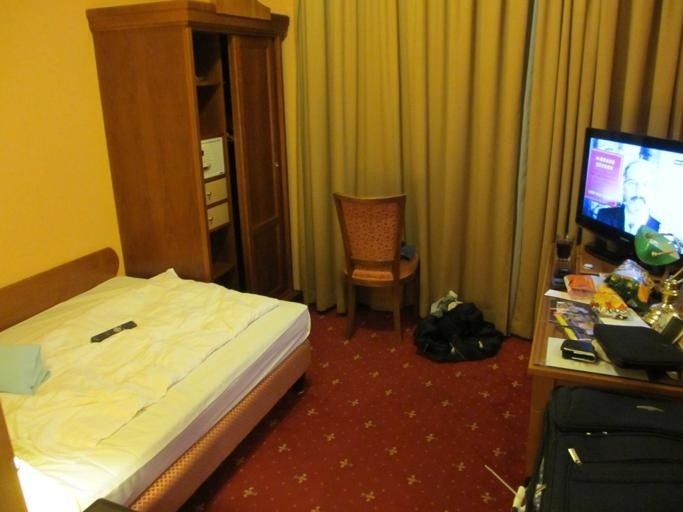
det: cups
[556,235,575,262]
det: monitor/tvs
[575,127,683,276]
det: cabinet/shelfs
[86,0,304,305]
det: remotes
[91,321,137,342]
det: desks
[522,245,683,507]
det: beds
[0,247,312,512]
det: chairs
[332,191,420,342]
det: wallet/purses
[560,339,598,363]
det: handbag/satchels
[593,324,683,372]
[411,302,505,363]
[523,384,683,510]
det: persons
[596,159,661,237]
